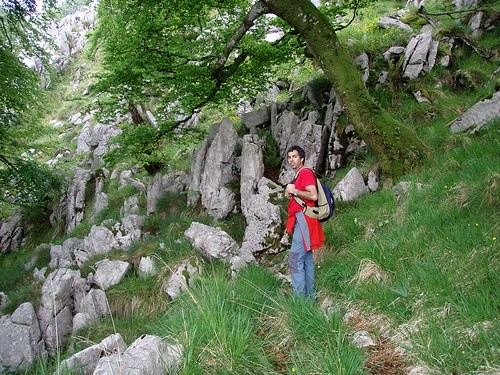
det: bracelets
[294,189,298,196]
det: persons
[285,146,326,300]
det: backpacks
[292,166,335,223]
